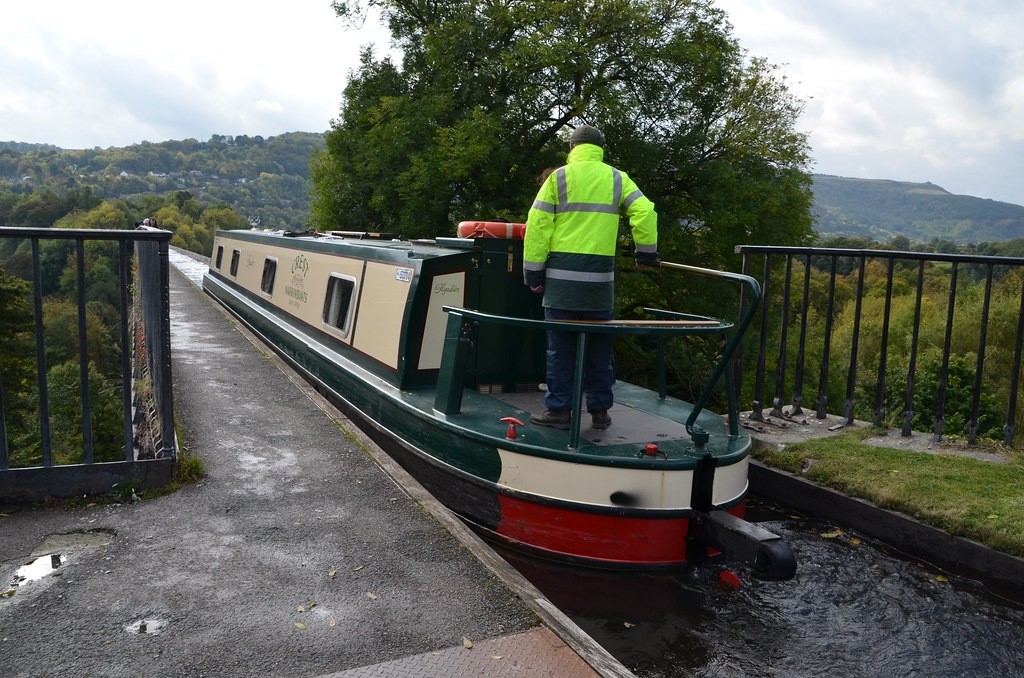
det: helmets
[570,125,606,148]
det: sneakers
[592,409,611,428]
[530,407,571,430]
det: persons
[520,125,661,430]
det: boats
[201,220,798,584]
